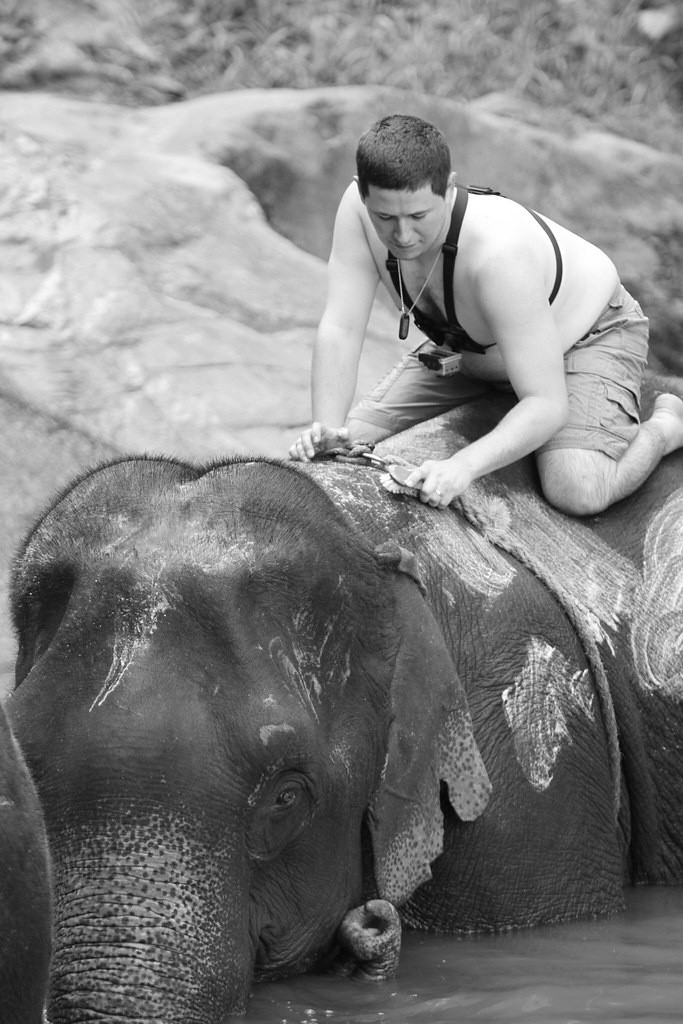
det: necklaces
[389,255,443,340]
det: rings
[435,488,444,498]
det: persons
[289,114,679,516]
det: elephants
[0,360,683,1023]
